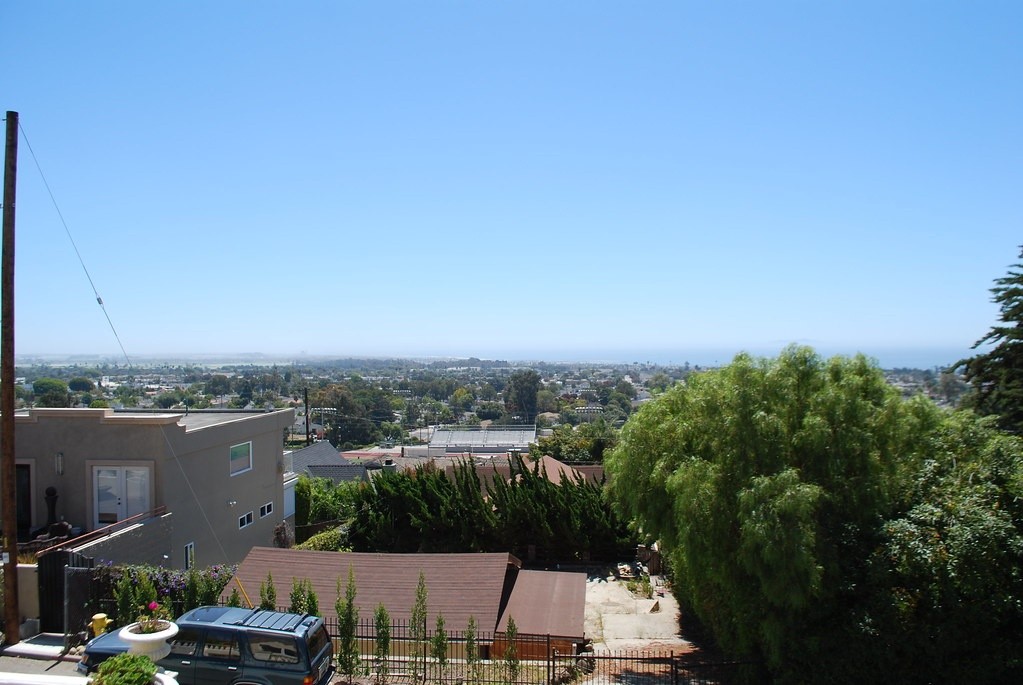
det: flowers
[134,602,172,633]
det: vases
[118,619,178,662]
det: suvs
[74,602,338,685]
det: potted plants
[19,616,39,639]
[86,651,179,685]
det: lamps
[228,501,237,508]
[55,453,64,476]
